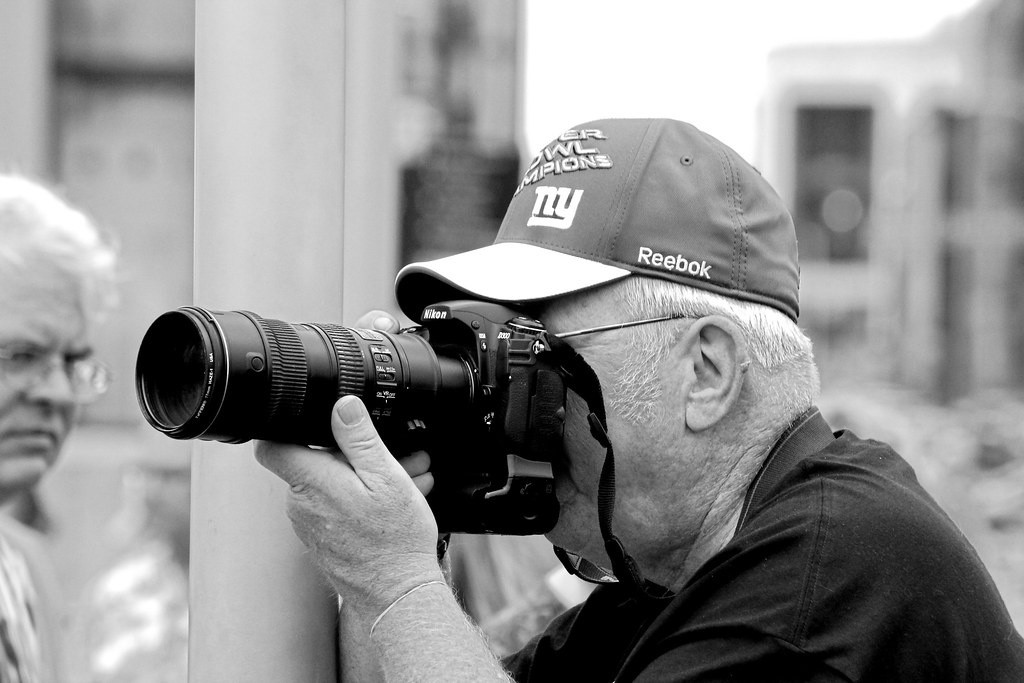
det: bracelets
[368,580,448,640]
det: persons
[256,120,1022,683]
[0,169,125,683]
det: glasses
[0,340,113,399]
[554,312,690,340]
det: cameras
[135,305,566,536]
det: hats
[395,119,799,326]
